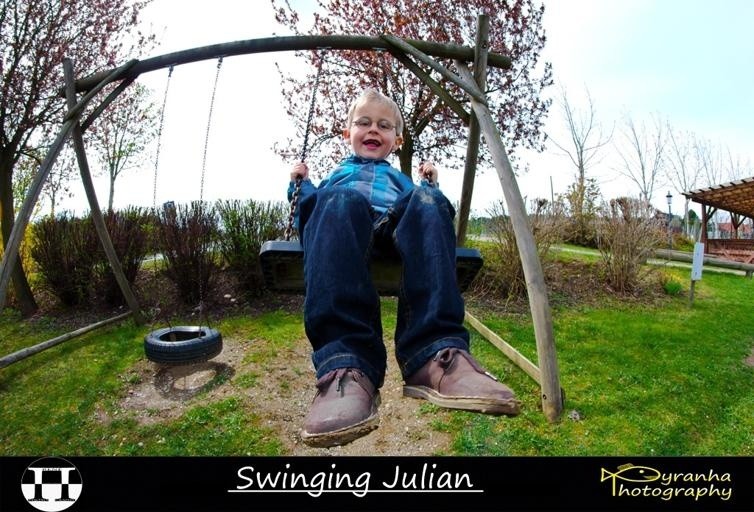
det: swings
[144,56,224,364]
[260,46,484,299]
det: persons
[287,90,522,449]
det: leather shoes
[401,348,522,418]
[298,366,382,449]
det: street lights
[665,189,674,266]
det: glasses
[348,115,399,132]
